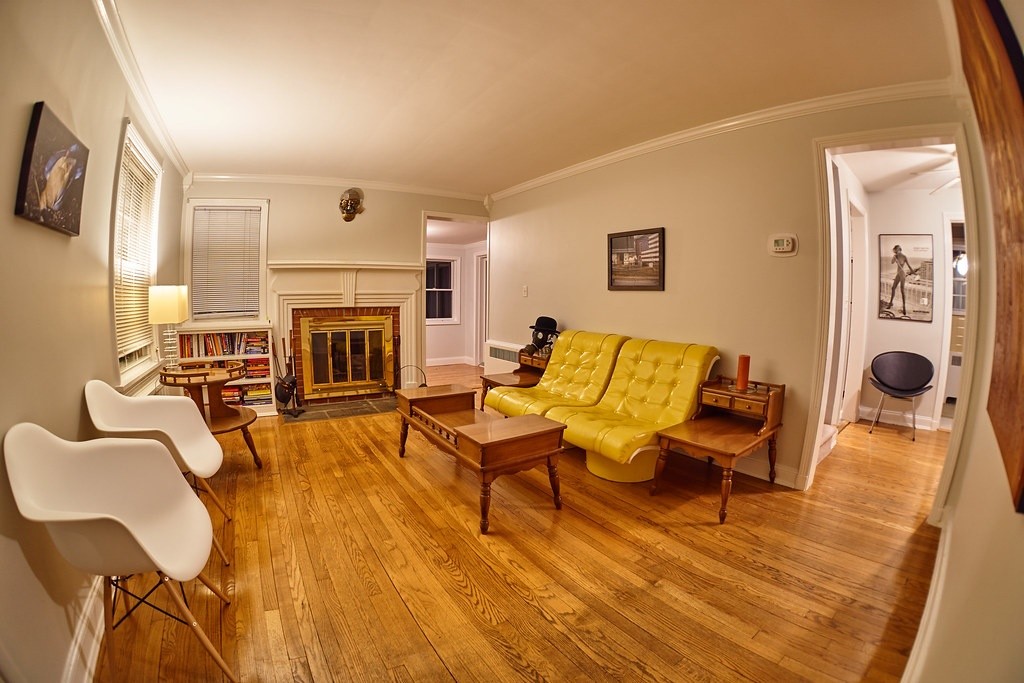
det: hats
[529,317,561,331]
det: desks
[478,370,542,420]
[203,403,263,468]
[645,409,784,526]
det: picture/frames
[878,233,934,322]
[607,227,666,292]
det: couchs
[484,329,632,420]
[549,337,721,484]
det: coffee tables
[394,383,568,537]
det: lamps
[147,284,190,372]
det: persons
[886,245,919,316]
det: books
[182,359,270,379]
[184,383,273,406]
[179,331,269,359]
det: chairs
[83,378,232,521]
[1,422,239,683]
[866,350,934,441]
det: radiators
[483,339,527,374]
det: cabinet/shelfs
[688,372,786,436]
[176,320,279,418]
[511,346,552,374]
